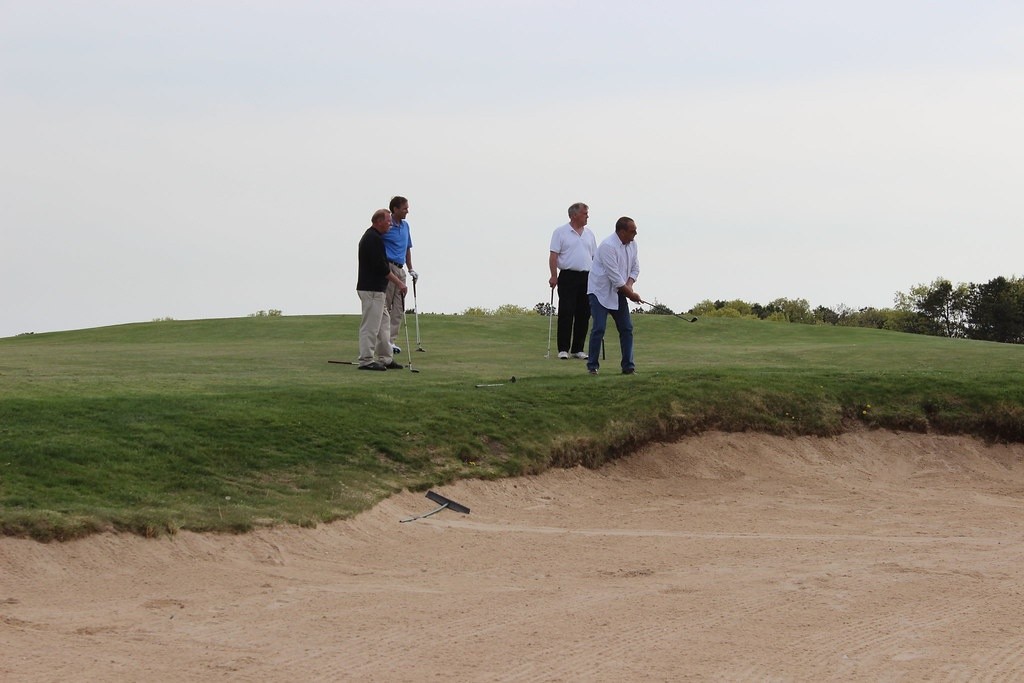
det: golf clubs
[328,360,359,365]
[412,275,426,352]
[640,299,698,323]
[601,337,606,360]
[399,291,420,373]
[475,376,517,388]
[544,288,553,360]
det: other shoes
[392,344,401,354]
[622,368,637,375]
[382,360,404,369]
[358,362,387,371]
[590,368,598,375]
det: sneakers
[558,351,568,359]
[572,352,589,359]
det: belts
[387,260,403,269]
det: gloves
[408,269,419,284]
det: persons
[357,209,407,371]
[385,196,418,353]
[586,217,644,375]
[549,203,597,359]
